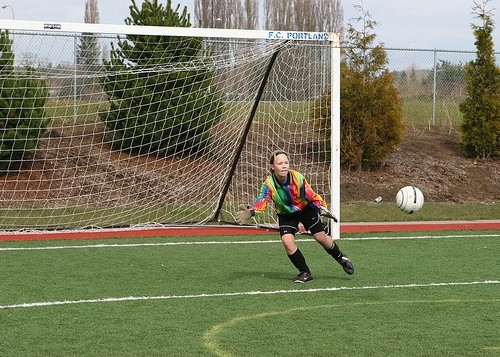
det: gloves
[319,206,339,224]
[233,203,252,226]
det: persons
[235,150,354,284]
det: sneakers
[293,271,314,284]
[336,253,355,276]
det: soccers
[396,186,424,214]
